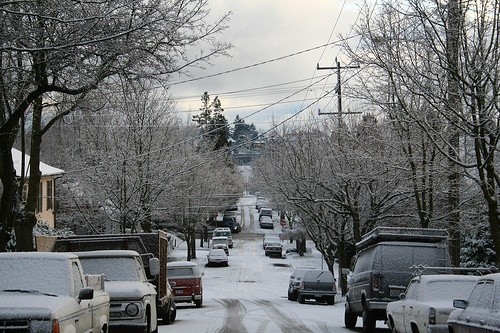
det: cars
[385,265,498,333]
[288,266,317,301]
[166,260,204,308]
[262,236,281,250]
[265,242,283,258]
[446,273,500,333]
[219,192,274,234]
[207,249,228,266]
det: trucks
[50,230,176,325]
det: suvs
[69,250,161,333]
[213,227,233,248]
[341,226,453,333]
[298,269,337,305]
[212,237,229,256]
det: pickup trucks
[0,252,111,333]
